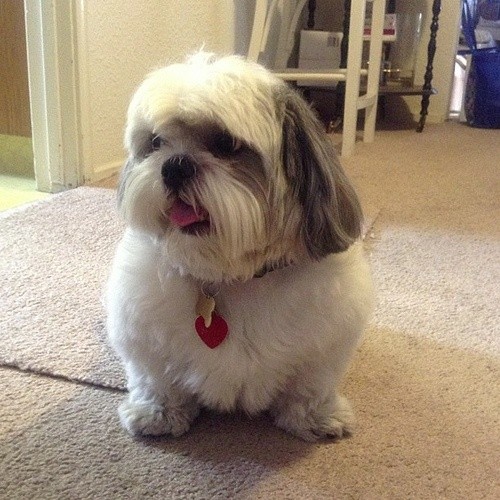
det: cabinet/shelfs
[248,0,386,157]
[303,0,441,132]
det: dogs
[105,51,380,443]
